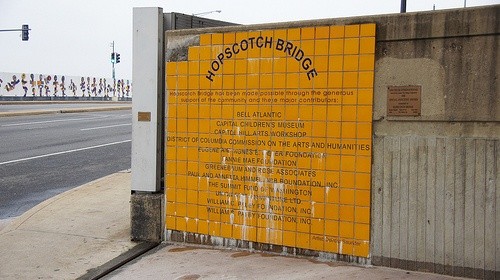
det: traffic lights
[115,53,120,63]
[111,53,114,63]
